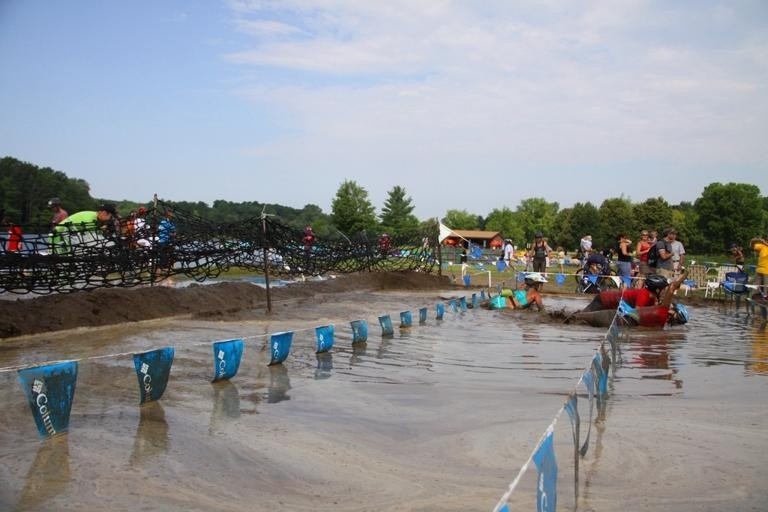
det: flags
[438,221,454,243]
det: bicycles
[573,256,617,284]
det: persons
[459,228,685,295]
[301,224,315,259]
[548,273,668,320]
[47,197,177,288]
[562,269,690,327]
[421,233,430,252]
[467,273,548,313]
[732,243,745,273]
[379,233,391,256]
[4,216,23,253]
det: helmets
[525,273,549,286]
[535,233,543,238]
[672,302,688,324]
[645,273,669,287]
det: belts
[673,260,679,262]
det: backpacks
[647,239,667,267]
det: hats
[99,204,118,215]
[663,228,678,235]
[47,198,62,208]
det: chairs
[675,266,749,298]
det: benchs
[719,281,768,333]
